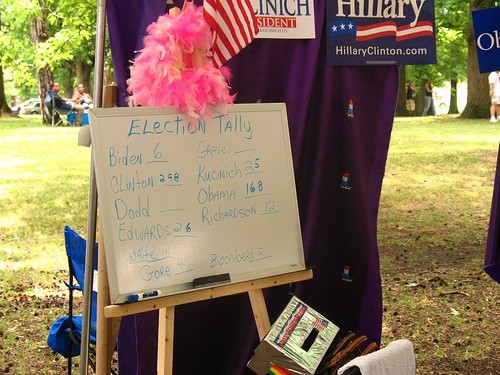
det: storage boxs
[246,294,340,375]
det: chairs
[42,91,76,127]
[47,226,118,375]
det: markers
[129,289,160,302]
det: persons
[488,70,500,122]
[44,82,84,126]
[73,84,92,109]
[422,78,433,115]
[406,80,417,115]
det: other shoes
[497,118,500,121]
[490,119,498,122]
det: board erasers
[192,273,232,288]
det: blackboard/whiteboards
[88,103,306,305]
